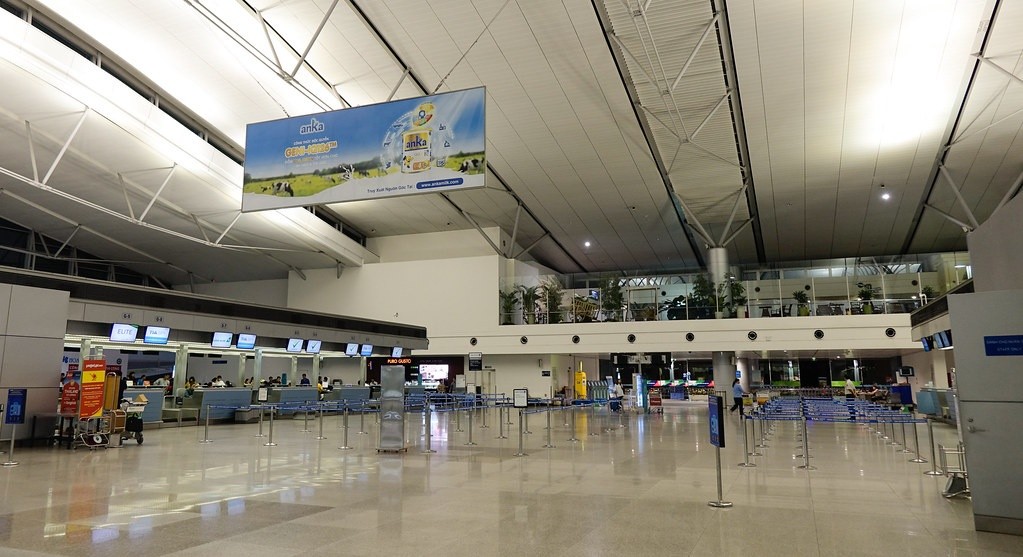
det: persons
[60,373,65,382]
[868,385,883,403]
[614,379,625,410]
[300,373,310,384]
[244,376,282,387]
[123,372,233,396]
[318,376,329,393]
[365,379,377,386]
[728,378,748,416]
[844,374,857,420]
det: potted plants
[665,275,748,319]
[628,302,662,322]
[541,284,563,324]
[516,285,541,324]
[602,275,624,322]
[858,289,874,314]
[793,290,810,316]
[499,290,518,325]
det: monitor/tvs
[211,331,234,348]
[286,338,304,352]
[921,331,951,352]
[360,344,373,356]
[346,342,359,356]
[143,325,171,344]
[236,333,257,349]
[305,339,322,353]
[392,346,403,358]
[109,323,139,343]
